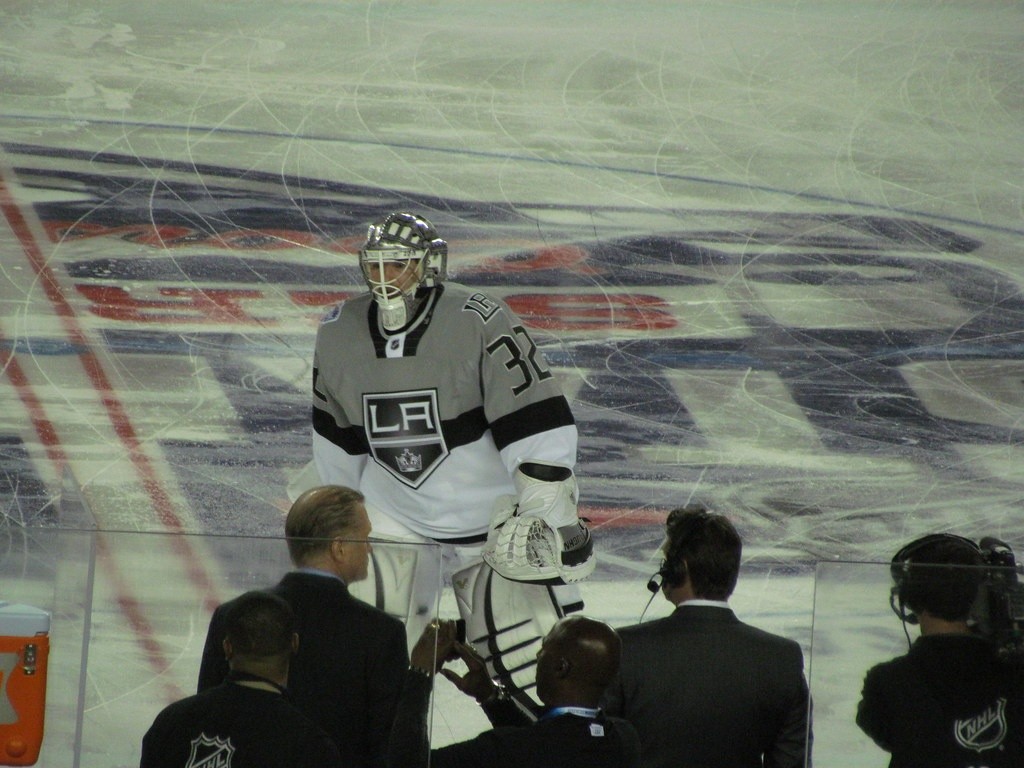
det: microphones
[889,587,918,625]
[647,567,669,593]
[979,536,1014,562]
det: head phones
[891,533,991,611]
[660,511,724,587]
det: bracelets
[409,666,434,678]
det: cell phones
[454,619,467,644]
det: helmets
[359,211,448,333]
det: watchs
[483,683,510,713]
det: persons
[385,616,638,768]
[603,505,814,768]
[193,485,410,768]
[311,213,596,730]
[856,535,1024,768]
[139,590,358,768]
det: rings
[473,648,479,654]
[431,623,440,632]
